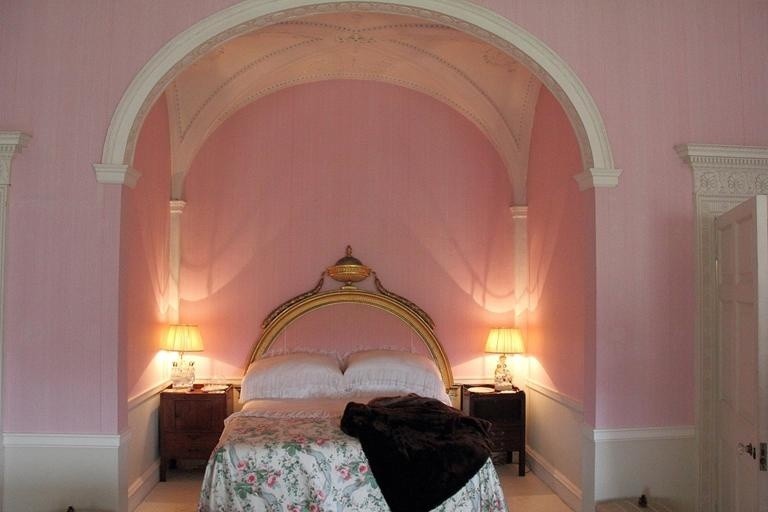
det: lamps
[158,324,204,391]
[484,326,526,390]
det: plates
[468,386,495,393]
[201,385,229,391]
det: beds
[198,243,509,512]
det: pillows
[239,351,452,406]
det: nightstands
[160,383,234,482]
[460,384,525,477]
